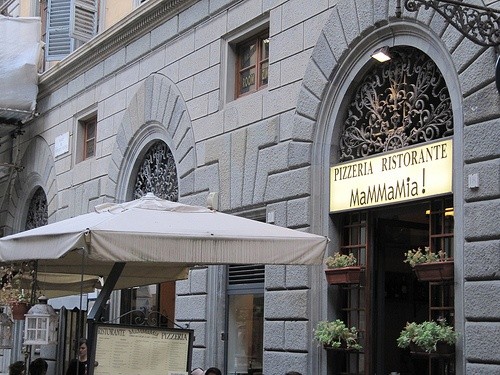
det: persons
[66,339,88,375]
[204,367,221,375]
[28,359,48,375]
[9,361,26,375]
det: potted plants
[313,319,362,351]
[325,252,360,284]
[396,321,461,354]
[404,247,454,281]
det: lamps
[370,46,391,62]
[23,294,57,345]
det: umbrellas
[0,268,98,303]
[0,193,331,375]
